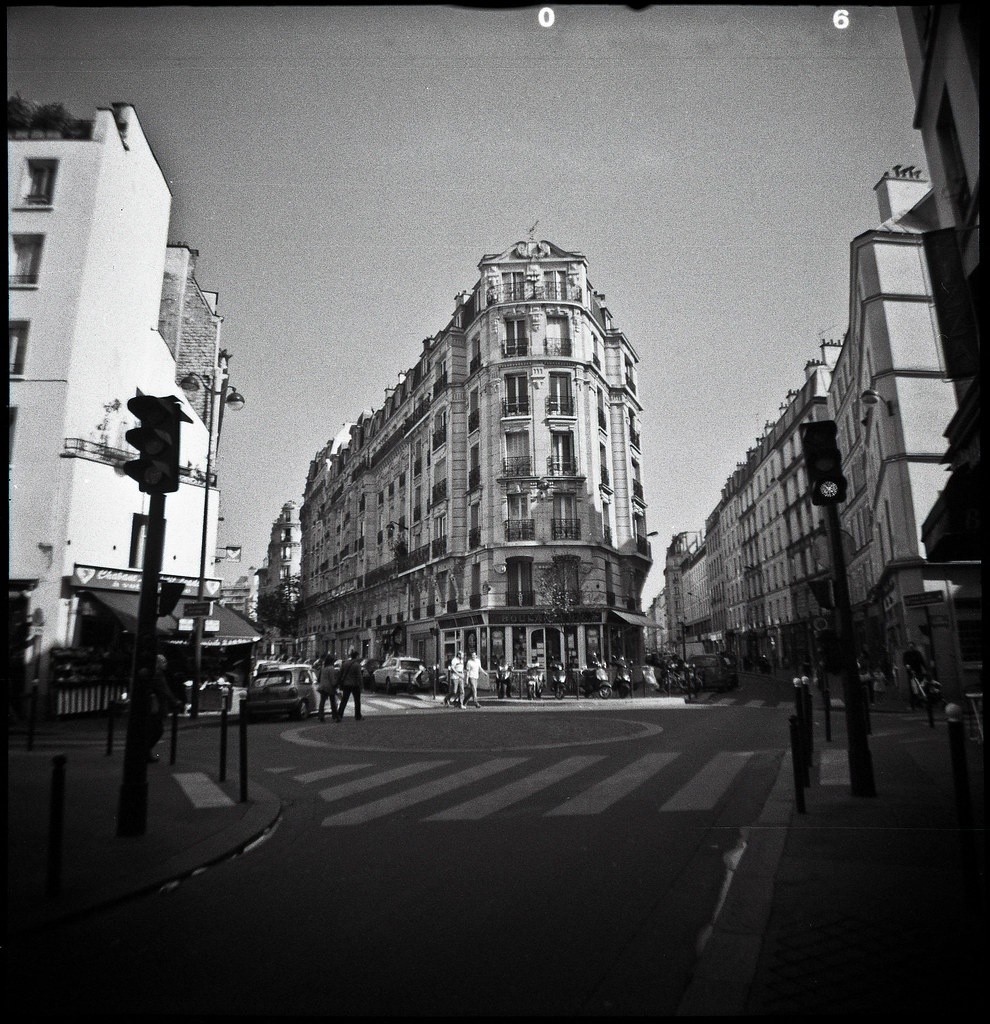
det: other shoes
[474,701,481,708]
[148,753,160,763]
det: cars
[333,658,383,689]
[245,667,332,717]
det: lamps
[820,479,838,498]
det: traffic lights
[159,581,184,617]
[123,396,181,491]
[809,576,831,609]
[799,419,848,505]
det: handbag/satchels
[339,680,344,690]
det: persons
[803,647,829,688]
[444,651,487,709]
[860,645,875,706]
[903,643,924,710]
[148,655,182,762]
[317,652,363,722]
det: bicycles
[662,660,704,695]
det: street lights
[180,372,245,717]
[688,592,713,632]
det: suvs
[373,658,427,694]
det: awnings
[612,609,664,629]
[90,590,264,646]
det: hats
[323,654,337,667]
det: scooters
[548,655,569,700]
[611,654,634,699]
[523,659,546,701]
[495,660,515,699]
[580,652,613,699]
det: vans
[691,654,737,691]
